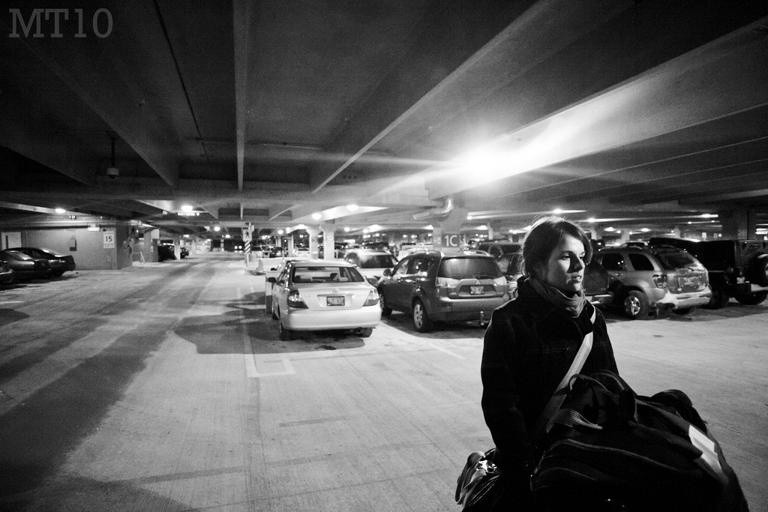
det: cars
[127,232,243,260]
[0,245,76,290]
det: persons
[480,215,618,511]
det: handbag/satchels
[454,447,528,511]
